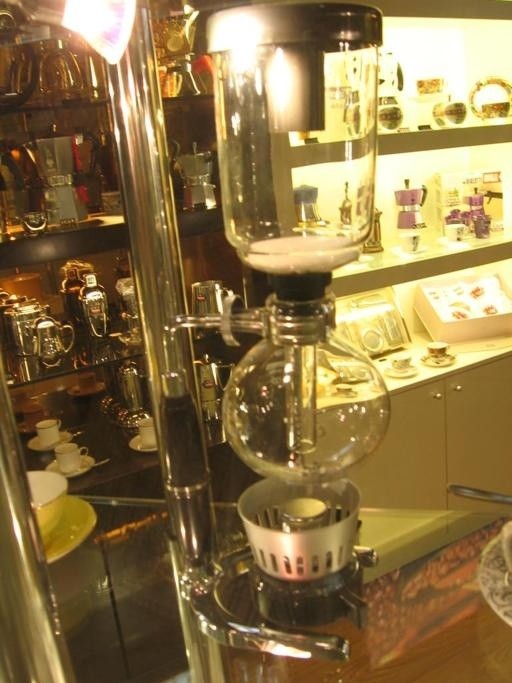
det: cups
[191,279,234,340]
[337,383,352,395]
[55,442,89,473]
[27,471,68,551]
[137,417,157,448]
[24,406,43,427]
[445,223,469,243]
[391,353,412,372]
[472,214,492,238]
[34,418,62,447]
[401,233,419,252]
[426,342,451,362]
[78,373,98,394]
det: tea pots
[23,122,100,184]
[192,352,236,426]
[168,137,216,212]
[32,316,75,369]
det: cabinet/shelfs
[1,3,512,682]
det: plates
[44,455,95,479]
[384,365,417,377]
[329,390,358,398]
[46,496,98,564]
[420,353,456,368]
[26,430,73,452]
[391,245,427,258]
[67,382,105,397]
[17,416,46,434]
[129,434,159,454]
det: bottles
[37,40,84,105]
[393,176,427,240]
[469,186,492,224]
[79,273,111,342]
[62,267,84,316]
